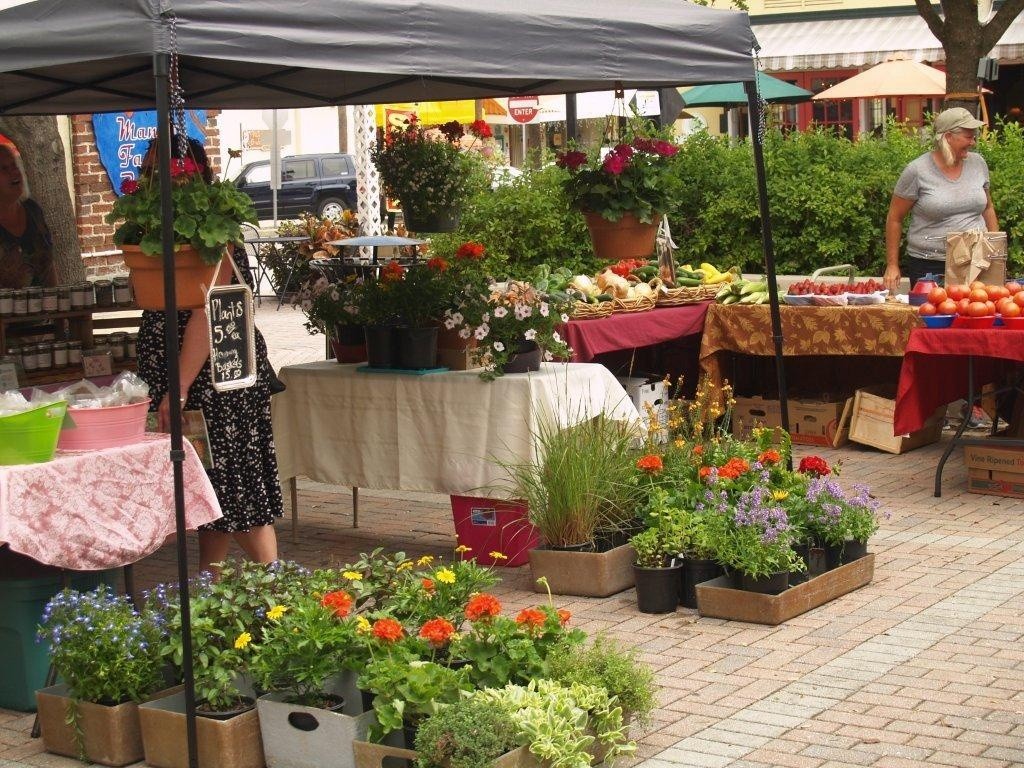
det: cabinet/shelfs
[0,305,144,388]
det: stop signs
[508,96,539,123]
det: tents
[0,0,794,768]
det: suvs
[230,153,387,224]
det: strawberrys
[788,278,886,297]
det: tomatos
[919,281,1024,319]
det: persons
[0,144,63,348]
[134,134,285,583]
[882,107,1000,290]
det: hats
[933,107,987,132]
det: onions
[572,268,652,300]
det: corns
[713,279,787,305]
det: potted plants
[453,354,680,598]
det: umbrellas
[680,71,815,108]
[811,60,994,99]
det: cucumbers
[629,260,702,287]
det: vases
[579,208,660,258]
[391,325,440,371]
[361,324,401,367]
[437,321,483,370]
[326,324,369,363]
[631,539,868,614]
[501,340,542,373]
[114,243,225,310]
[402,206,464,233]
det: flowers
[367,108,686,224]
[627,372,891,581]
[32,553,588,765]
[290,240,576,383]
[106,149,261,267]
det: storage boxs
[614,370,1024,499]
[449,491,539,567]
[695,552,875,625]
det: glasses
[136,166,156,178]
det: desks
[242,236,311,311]
[559,301,928,420]
[894,327,1024,496]
[0,430,224,738]
[269,363,648,542]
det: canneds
[0,276,142,373]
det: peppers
[607,259,645,277]
[526,264,572,298]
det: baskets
[626,275,706,305]
[564,283,616,318]
[704,284,730,299]
[613,278,662,311]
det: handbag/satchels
[267,359,287,394]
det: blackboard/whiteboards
[207,285,258,392]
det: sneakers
[966,406,993,428]
[997,417,1009,428]
